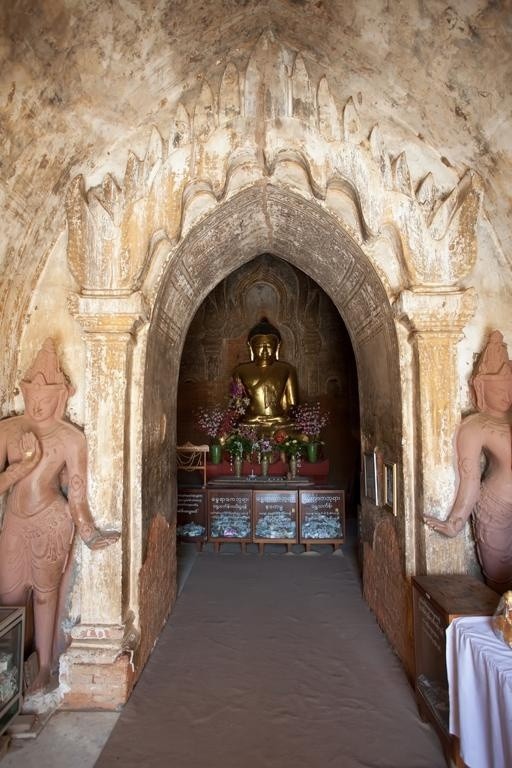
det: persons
[214,316,311,447]
[0,337,120,695]
[422,328,511,594]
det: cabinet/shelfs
[408,571,511,767]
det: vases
[210,443,318,478]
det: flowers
[192,392,331,457]
